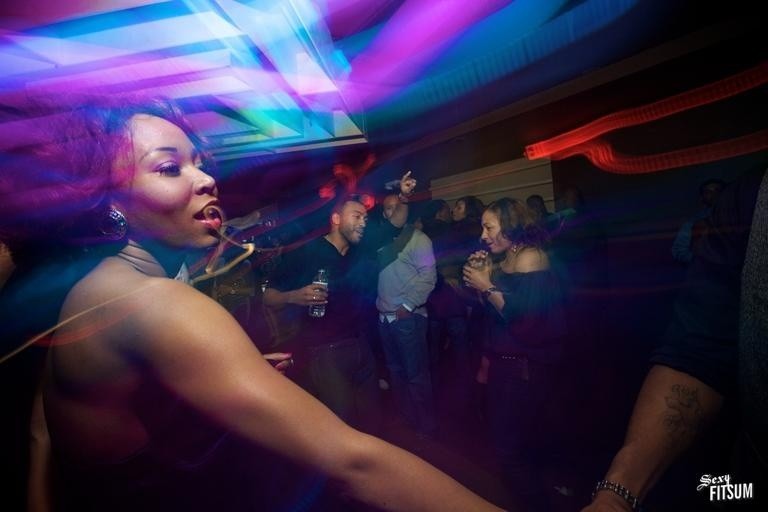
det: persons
[0,91,767,512]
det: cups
[469,255,488,274]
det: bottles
[307,268,331,318]
